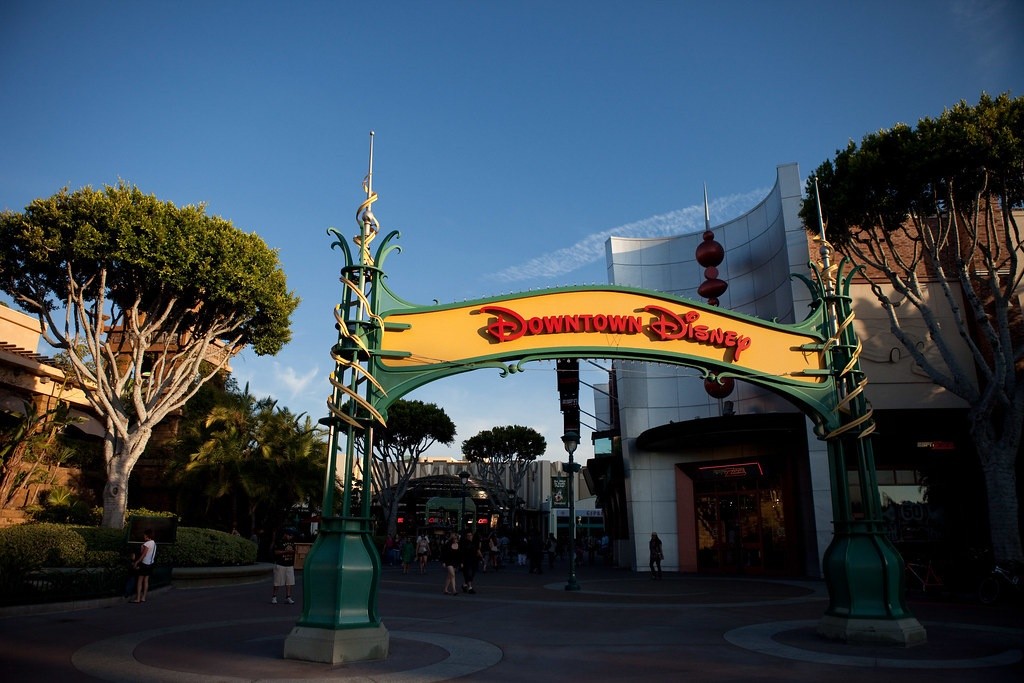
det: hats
[285,527,298,535]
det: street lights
[458,470,472,544]
[560,432,586,598]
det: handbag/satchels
[140,566,150,576]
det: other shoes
[286,597,294,604]
[271,597,277,604]
[444,591,451,594]
[462,584,469,593]
[468,588,476,594]
[129,599,139,603]
[453,592,458,596]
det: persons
[272,529,297,605]
[384,531,609,596]
[650,532,662,580]
[130,529,157,602]
